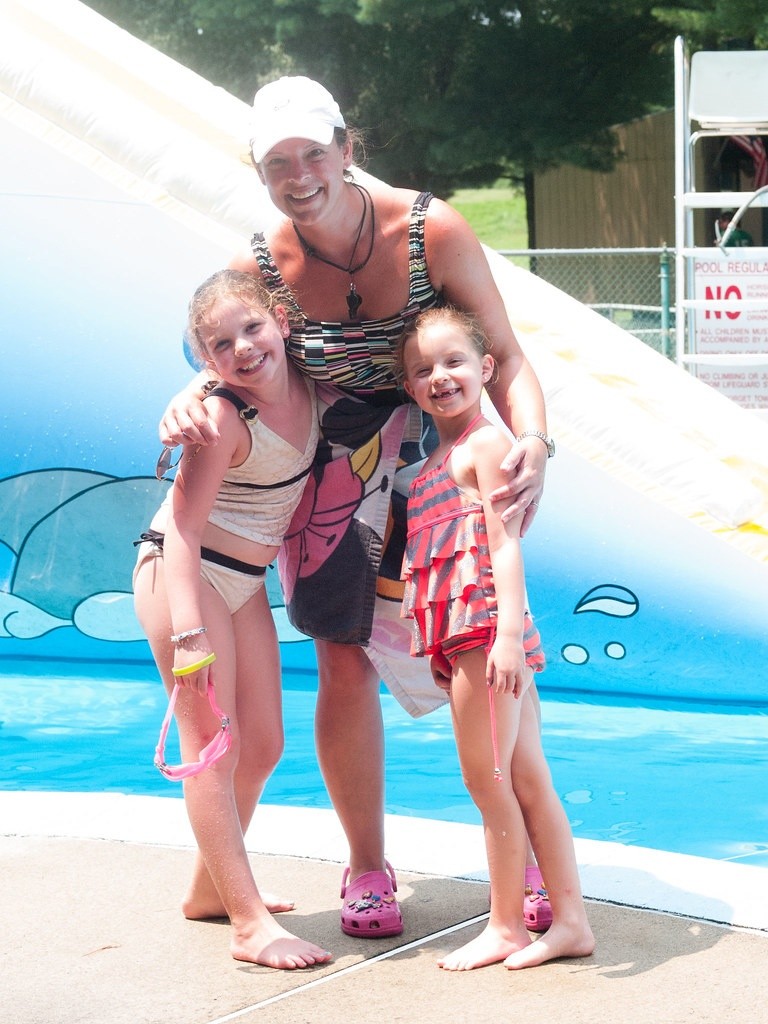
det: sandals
[340,859,403,938]
[488,865,553,931]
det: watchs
[516,431,555,459]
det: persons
[158,76,556,937]
[131,269,332,969]
[714,212,754,246]
[401,307,594,971]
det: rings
[531,501,539,507]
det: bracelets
[172,653,216,676]
[170,628,207,642]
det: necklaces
[293,181,375,319]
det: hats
[252,75,345,164]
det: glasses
[162,720,234,782]
